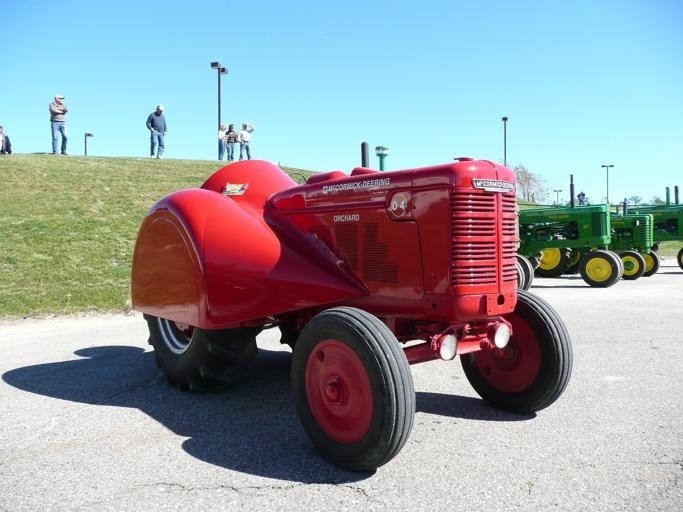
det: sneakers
[151,154,163,159]
[53,150,68,155]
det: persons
[225,124,239,162]
[577,191,586,205]
[0,126,12,154]
[237,121,255,161]
[146,104,168,159]
[218,123,230,160]
[49,95,68,156]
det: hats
[242,123,247,126]
[157,105,164,111]
[55,96,64,99]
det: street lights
[503,116,508,166]
[601,165,614,204]
[554,189,562,204]
[375,146,389,170]
[85,132,93,156]
[211,62,228,159]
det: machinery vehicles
[519,203,683,292]
[130,156,574,471]
